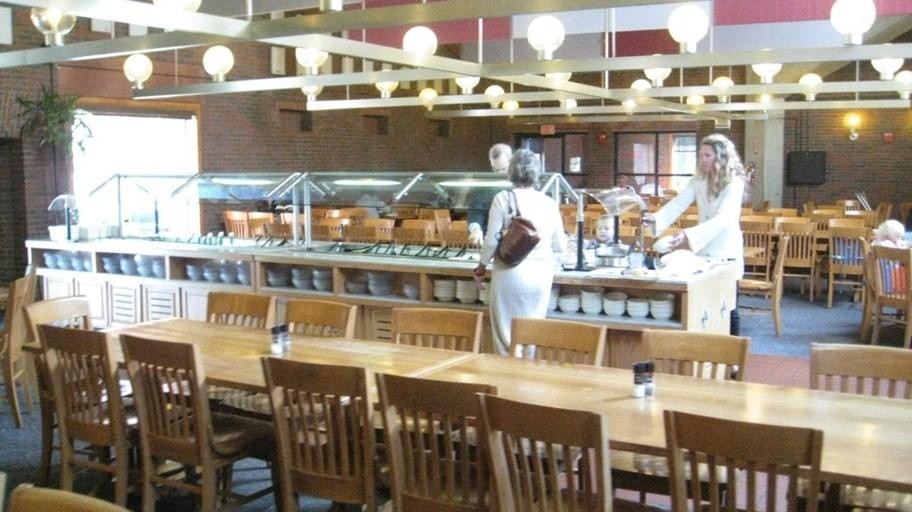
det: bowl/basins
[120,257,135,274]
[101,257,118,273]
[185,263,202,279]
[236,267,252,283]
[265,267,287,287]
[71,256,84,270]
[654,234,676,255]
[604,292,626,316]
[151,260,165,277]
[549,285,560,312]
[366,271,393,296]
[135,257,150,275]
[627,299,649,318]
[219,266,235,281]
[291,267,312,290]
[479,282,492,306]
[434,279,456,301]
[580,287,603,315]
[455,279,478,303]
[558,295,580,313]
[312,268,332,290]
[202,265,218,280]
[45,255,56,267]
[402,284,420,298]
[649,293,676,320]
[84,260,92,270]
[57,255,70,269]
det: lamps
[201,44,235,82]
[527,15,565,61]
[829,0,876,46]
[123,53,153,89]
[375,69,398,99]
[418,87,438,112]
[758,94,774,114]
[666,5,710,54]
[560,98,577,116]
[644,53,671,87]
[751,48,782,84]
[712,75,734,102]
[870,42,904,80]
[28,6,76,47]
[631,78,651,102]
[501,101,519,118]
[484,84,505,108]
[622,99,635,114]
[842,111,862,142]
[300,85,324,100]
[799,73,823,101]
[294,48,329,74]
[894,70,912,100]
[545,58,573,83]
[686,95,705,106]
[401,24,439,56]
[454,75,481,94]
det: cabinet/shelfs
[24,167,738,379]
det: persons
[595,215,622,247]
[616,174,635,194]
[641,132,747,377]
[466,143,513,247]
[869,217,905,293]
[472,148,566,360]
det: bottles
[281,324,291,353]
[645,361,655,395]
[631,362,646,398]
[270,326,283,354]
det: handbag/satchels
[495,187,541,266]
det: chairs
[0,272,37,429]
[577,326,750,505]
[737,234,789,337]
[774,216,810,233]
[827,217,865,229]
[325,208,349,219]
[817,204,843,218]
[777,220,818,302]
[415,208,437,221]
[222,210,249,238]
[768,207,798,217]
[301,224,330,241]
[786,341,912,512]
[390,227,428,246]
[267,223,294,239]
[344,206,368,221]
[871,243,912,349]
[341,224,379,245]
[858,235,902,344]
[885,202,894,220]
[451,219,468,229]
[358,305,484,443]
[311,207,326,222]
[20,293,133,482]
[811,212,835,230]
[218,296,358,504]
[836,198,862,211]
[901,202,912,223]
[432,208,452,234]
[281,211,304,225]
[660,408,824,512]
[741,215,773,222]
[247,211,275,238]
[401,219,437,235]
[362,217,396,234]
[319,217,352,238]
[395,207,418,217]
[740,220,773,300]
[827,225,872,308]
[35,323,193,508]
[374,371,548,512]
[442,228,479,249]
[872,203,883,227]
[474,392,664,512]
[118,333,282,512]
[259,355,431,512]
[451,317,609,476]
[160,290,277,485]
